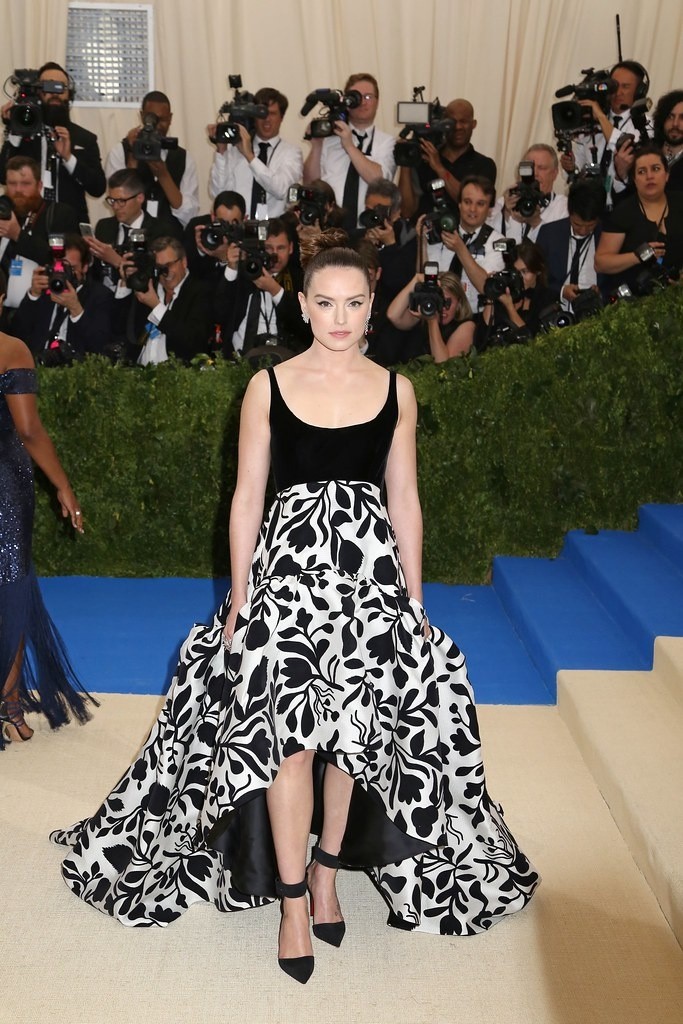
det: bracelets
[75,512,80,516]
[442,171,451,180]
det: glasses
[105,193,139,207]
[166,257,181,270]
[363,94,378,102]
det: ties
[449,234,469,284]
[46,304,65,351]
[342,130,368,231]
[242,289,262,353]
[600,116,622,168]
[121,225,132,245]
[43,130,56,201]
[570,235,589,285]
[250,143,271,221]
[164,290,175,306]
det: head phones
[67,74,76,100]
[632,64,650,101]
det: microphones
[555,84,574,98]
[301,100,319,115]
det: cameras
[125,228,156,292]
[614,97,654,152]
[201,161,678,335]
[40,233,76,292]
[0,194,12,220]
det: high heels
[0,700,34,742]
[308,846,345,948]
[278,873,314,984]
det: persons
[0,60,683,366]
[0,267,83,751]
[222,231,430,983]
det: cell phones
[81,223,94,240]
